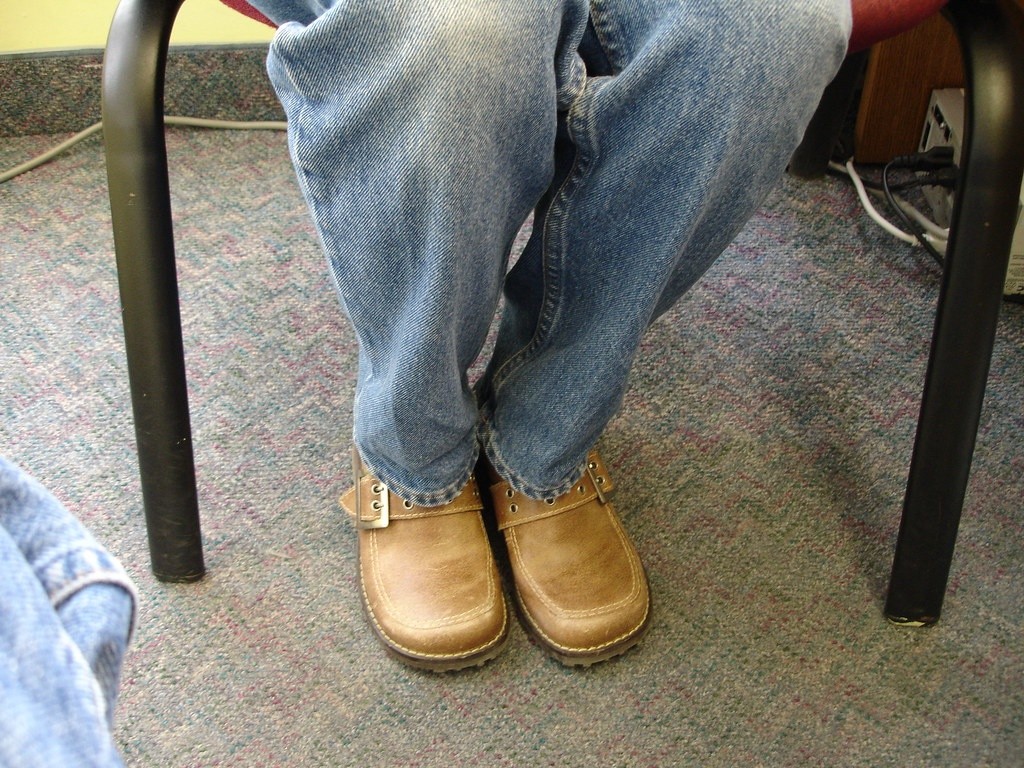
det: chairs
[100,0,1024,630]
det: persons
[238,0,853,673]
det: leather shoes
[489,447,654,668]
[339,445,512,672]
